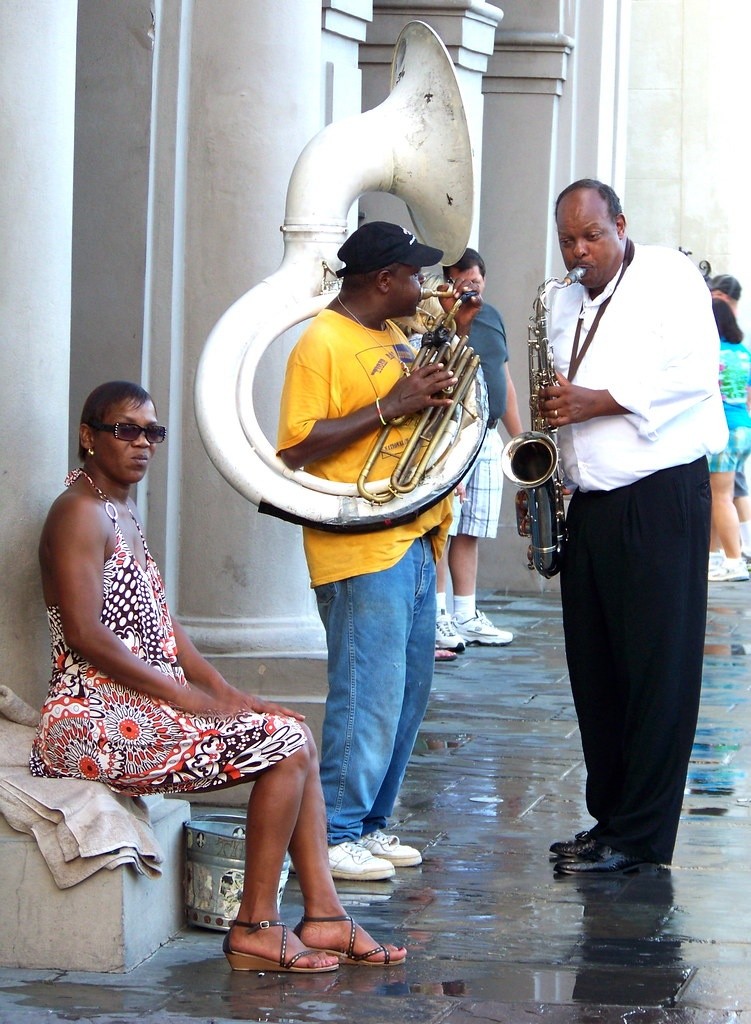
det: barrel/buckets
[182,813,290,932]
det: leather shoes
[555,842,661,875]
[550,832,605,857]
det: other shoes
[435,647,456,661]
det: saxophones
[498,262,591,584]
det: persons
[515,178,729,875]
[708,273,751,583]
[390,248,524,660]
[28,381,408,974]
[277,221,454,881]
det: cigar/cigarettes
[463,497,472,502]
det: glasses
[89,423,167,443]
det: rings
[554,409,558,418]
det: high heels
[293,915,407,966]
[223,920,340,973]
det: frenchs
[192,18,493,536]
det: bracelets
[376,397,387,425]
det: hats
[707,276,740,299]
[335,220,445,278]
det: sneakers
[291,839,396,881]
[357,830,422,867]
[435,616,465,650]
[453,609,514,645]
[706,554,750,582]
